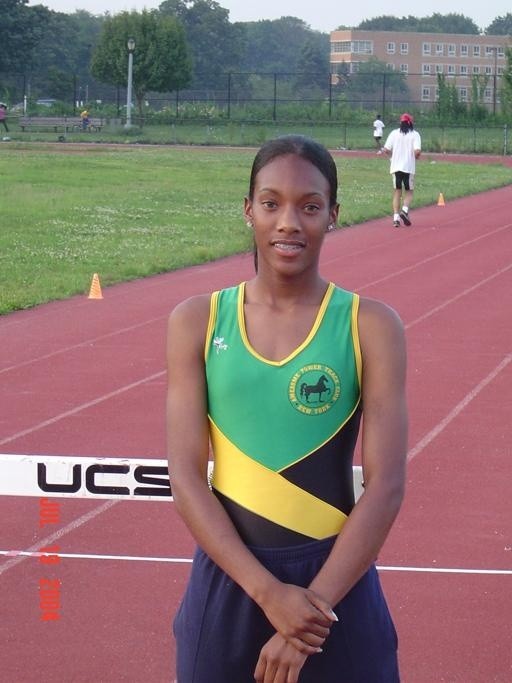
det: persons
[80,110,89,129]
[0,105,10,132]
[163,136,406,682]
[384,113,423,228]
[373,113,385,154]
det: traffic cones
[437,191,446,207]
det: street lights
[85,269,105,300]
[122,37,135,127]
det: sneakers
[376,147,384,155]
[393,219,400,227]
[399,209,412,226]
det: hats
[399,112,414,126]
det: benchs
[63,117,106,132]
[16,116,67,132]
